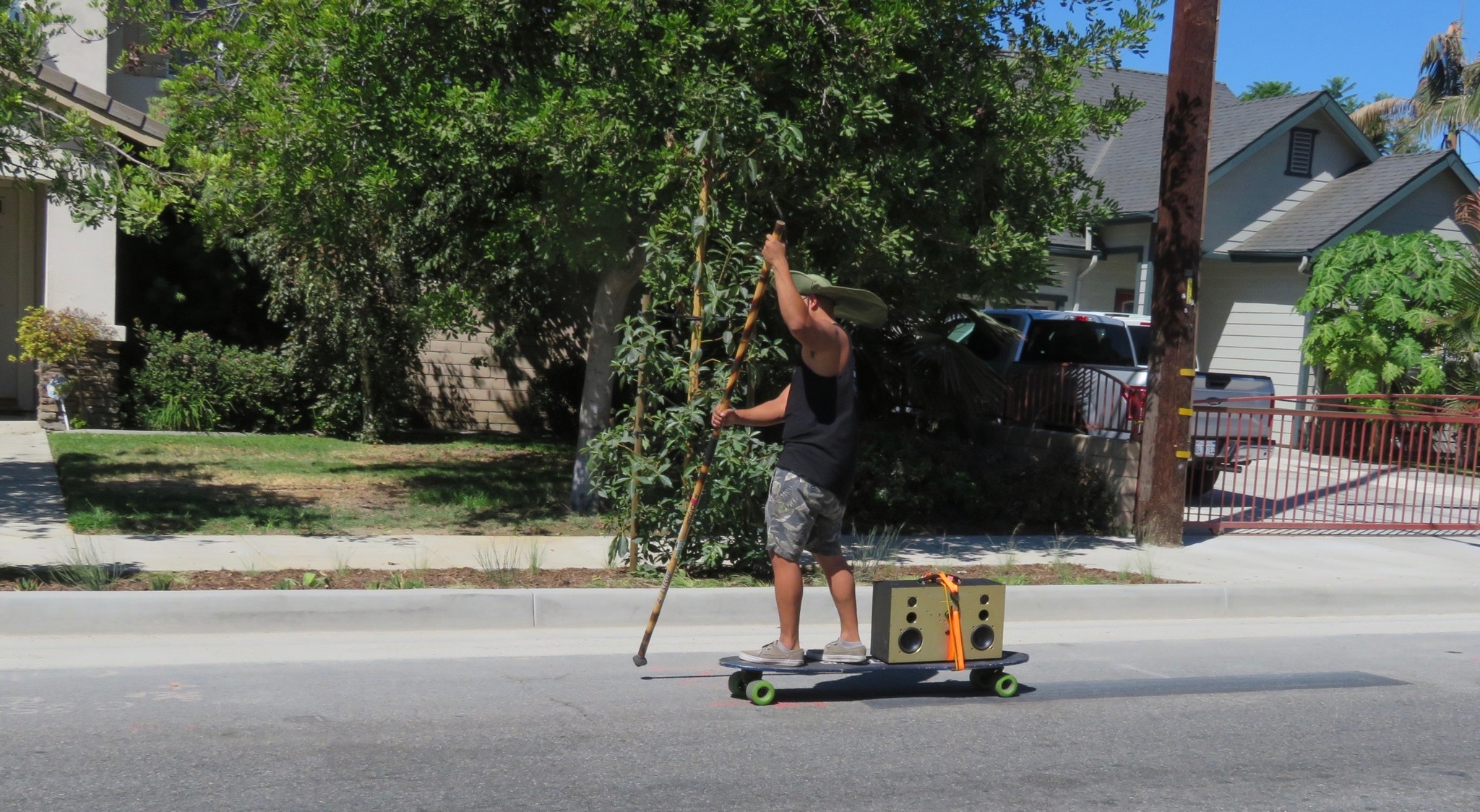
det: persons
[711,233,889,667]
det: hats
[769,271,888,328]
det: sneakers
[739,639,804,666]
[806,639,866,662]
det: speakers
[869,578,1006,662]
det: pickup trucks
[890,307,1277,497]
